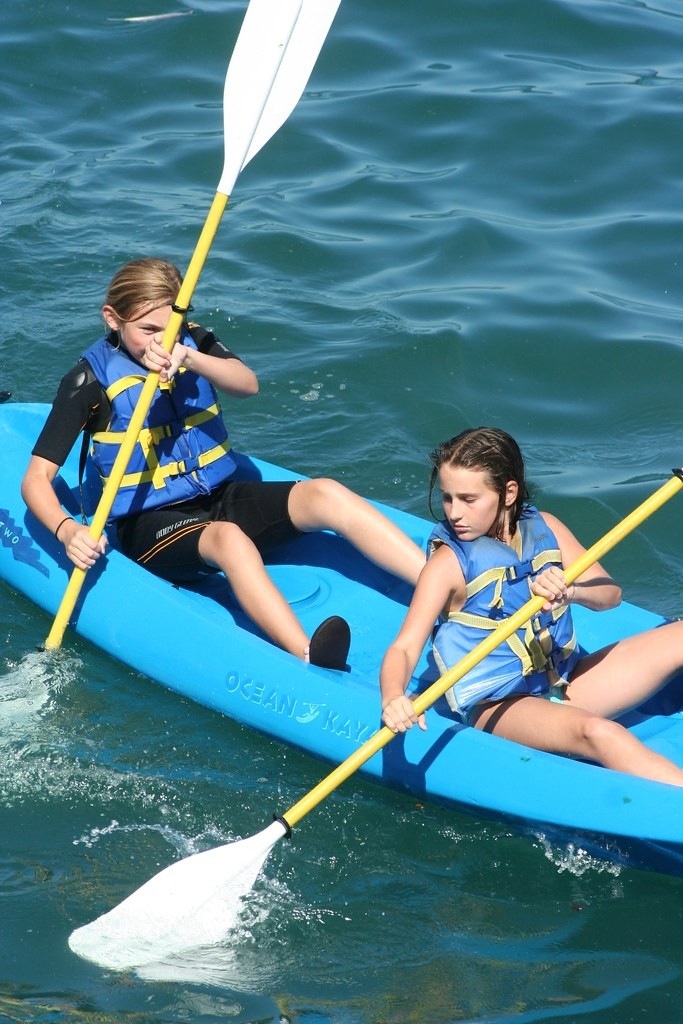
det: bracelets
[55,517,73,543]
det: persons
[21,258,427,669]
[380,425,683,786]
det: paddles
[39,0,346,656]
[63,466,683,974]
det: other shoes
[308,616,351,671]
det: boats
[0,387,683,843]
